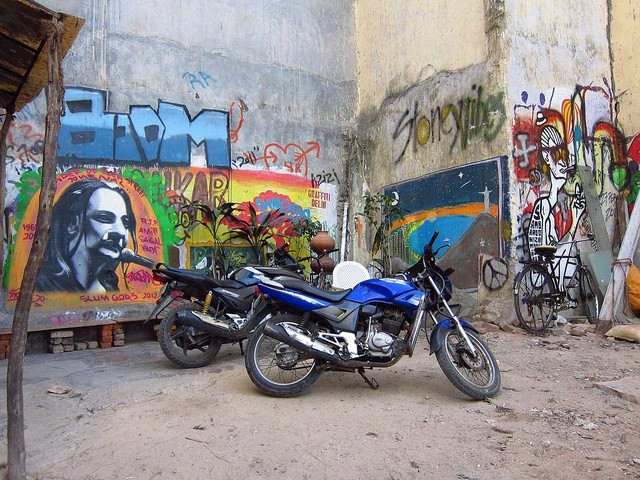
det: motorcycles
[144,242,333,368]
[240,228,501,400]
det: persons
[35,178,139,293]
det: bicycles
[290,248,340,292]
[511,233,602,335]
